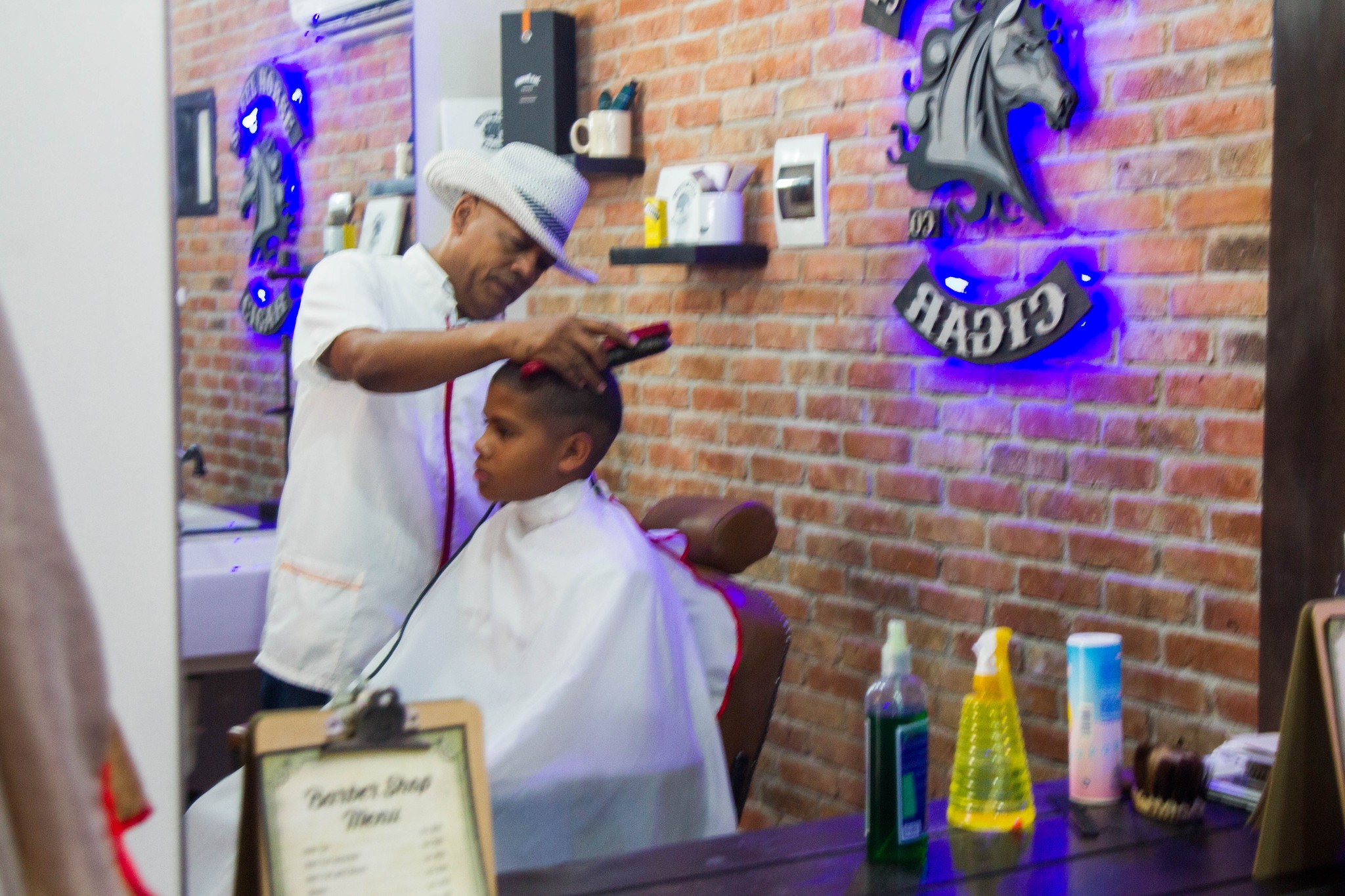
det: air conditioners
[289,0,413,48]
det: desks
[495,768,1257,896]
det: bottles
[1065,632,1123,806]
[598,80,636,110]
[946,627,1036,834]
[864,620,929,874]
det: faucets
[177,443,212,480]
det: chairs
[226,494,792,832]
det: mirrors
[162,0,1277,896]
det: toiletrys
[861,617,931,861]
[943,620,1037,836]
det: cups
[695,192,743,244]
[395,143,414,177]
[322,223,356,255]
[569,110,631,157]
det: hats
[426,142,602,287]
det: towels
[1,290,126,893]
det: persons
[180,356,737,896]
[251,141,638,711]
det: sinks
[176,527,282,655]
[181,501,255,531]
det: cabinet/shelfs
[555,147,768,270]
